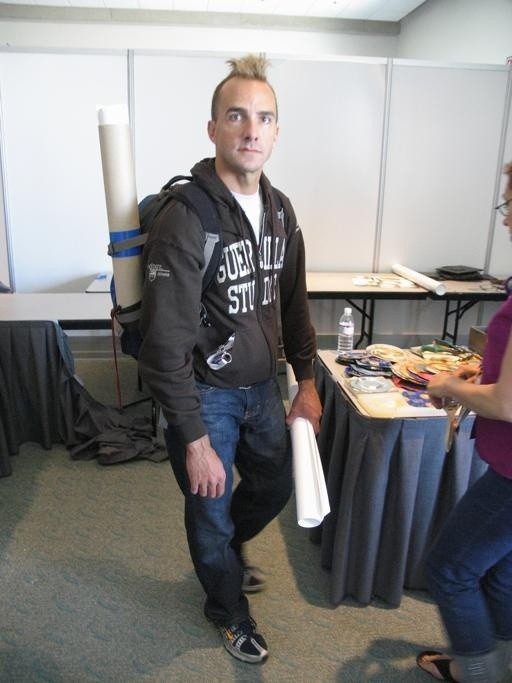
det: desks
[314,347,485,608]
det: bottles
[337,307,355,356]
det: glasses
[495,198,511,216]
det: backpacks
[111,174,225,359]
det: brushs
[442,396,463,453]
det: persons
[415,155,511,682]
[139,71,325,664]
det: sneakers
[218,614,269,664]
[241,560,266,592]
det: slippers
[417,650,464,682]
